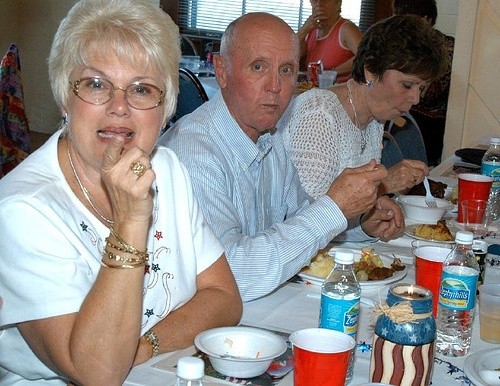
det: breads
[412,219,454,241]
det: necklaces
[347,79,371,153]
[66,134,114,223]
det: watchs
[144,330,160,357]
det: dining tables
[115,154,500,386]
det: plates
[404,222,490,243]
[292,82,313,92]
[297,248,407,286]
[464,347,500,386]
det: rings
[131,161,147,175]
[411,175,417,183]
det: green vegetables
[355,248,375,275]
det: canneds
[307,60,324,87]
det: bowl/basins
[398,194,454,223]
[194,327,289,377]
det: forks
[423,176,438,207]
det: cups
[461,199,489,240]
[318,70,339,87]
[289,327,356,386]
[412,240,500,345]
[458,173,495,223]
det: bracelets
[100,227,150,270]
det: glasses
[70,75,164,112]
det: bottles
[318,249,361,385]
[482,137,500,220]
[434,230,480,356]
[369,282,437,386]
[175,356,205,386]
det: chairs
[381,131,404,169]
[164,66,209,132]
[387,111,429,167]
[0,42,36,181]
[180,36,201,77]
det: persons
[395,0,455,115]
[298,0,364,85]
[279,12,447,200]
[0,0,243,386]
[157,12,405,302]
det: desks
[180,54,339,102]
